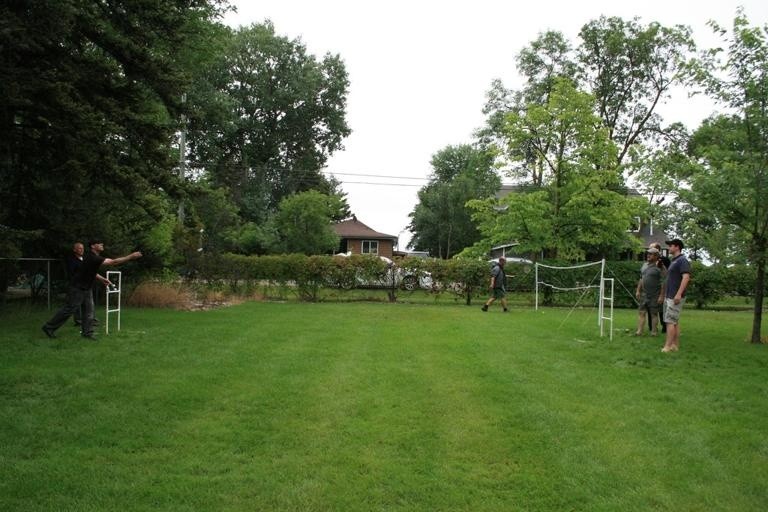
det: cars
[323,254,438,290]
[465,257,540,294]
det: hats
[645,248,660,257]
[665,239,683,249]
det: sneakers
[42,325,56,339]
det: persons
[633,248,664,337]
[646,242,671,333]
[66,242,101,327]
[659,239,691,353]
[41,238,143,341]
[481,255,511,313]
